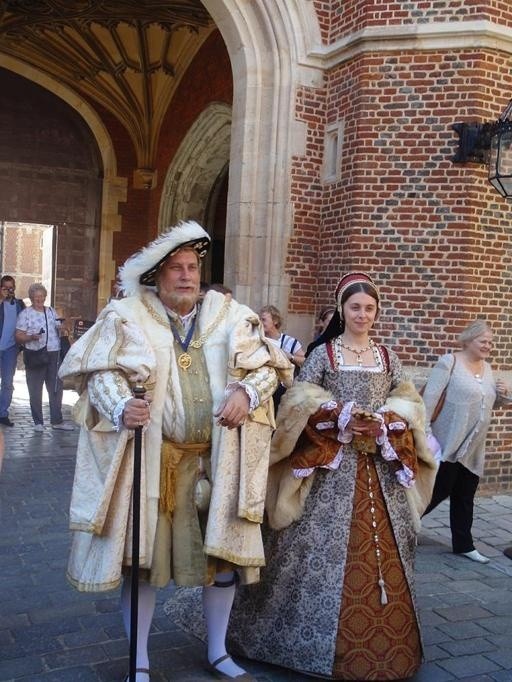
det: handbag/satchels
[273,333,297,406]
[418,353,457,423]
[23,348,49,369]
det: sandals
[124,667,151,682]
[205,653,258,682]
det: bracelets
[289,353,295,362]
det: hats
[114,220,211,296]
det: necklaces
[166,314,197,370]
[473,367,483,379]
[337,334,376,364]
[198,281,209,303]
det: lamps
[450,97,512,198]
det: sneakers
[461,550,491,564]
[35,424,74,432]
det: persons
[209,283,233,302]
[0,274,29,428]
[420,320,512,564]
[164,268,443,680]
[259,305,306,419]
[57,217,299,680]
[16,283,76,431]
[313,307,336,342]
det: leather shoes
[0,417,14,426]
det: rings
[238,420,244,426]
[352,427,356,431]
[219,415,225,422]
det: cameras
[39,328,45,334]
[3,287,14,294]
